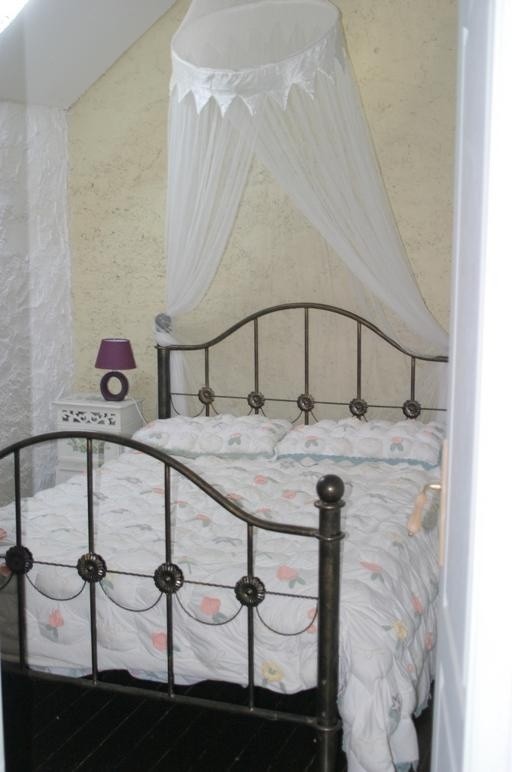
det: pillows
[273,415,448,471]
[125,416,294,461]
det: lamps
[93,338,137,402]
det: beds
[1,299,452,772]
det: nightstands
[50,390,145,487]
